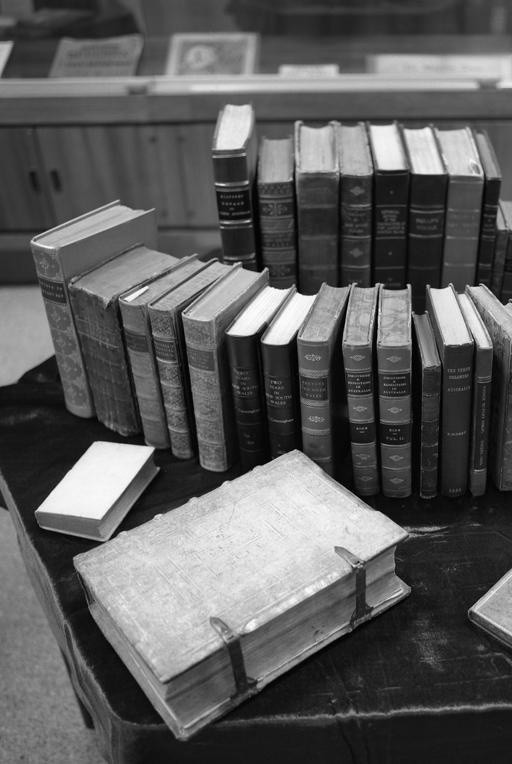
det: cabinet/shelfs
[0,0,512,286]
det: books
[225,281,297,473]
[148,257,239,460]
[256,135,297,290]
[73,448,413,742]
[401,121,448,312]
[426,283,474,498]
[367,121,410,290]
[181,263,270,472]
[501,200,512,302]
[458,290,493,497]
[343,284,379,497]
[434,124,484,289]
[467,281,512,493]
[337,120,372,287]
[297,282,351,484]
[212,103,258,271]
[292,120,340,289]
[467,567,511,647]
[260,284,320,459]
[474,127,501,289]
[493,208,508,298]
[411,310,441,499]
[118,253,218,449]
[67,241,190,439]
[377,282,413,499]
[35,441,159,544]
[29,198,157,419]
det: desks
[0,249,512,764]
